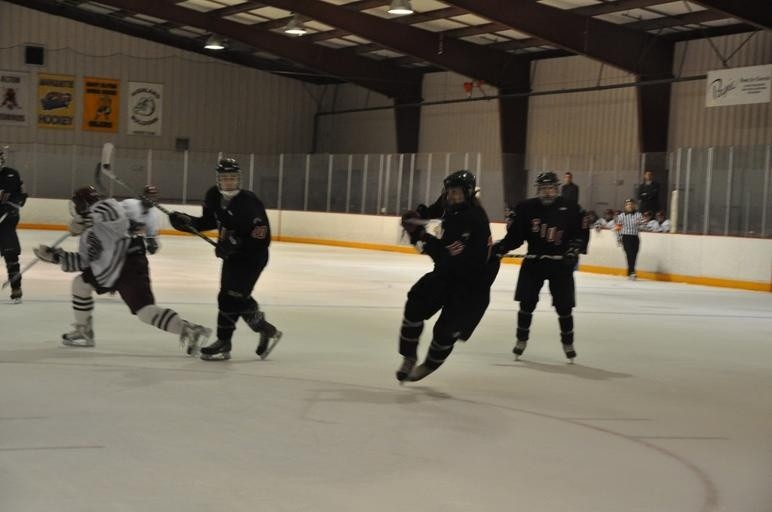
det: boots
[253,319,277,356]
[395,357,414,381]
[561,342,577,359]
[200,338,232,354]
[184,319,204,355]
[10,287,23,299]
[61,315,95,341]
[511,338,528,356]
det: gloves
[33,243,61,265]
[401,204,429,233]
[560,248,582,268]
[146,238,158,254]
[168,212,192,230]
[214,234,241,258]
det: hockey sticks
[101,143,218,246]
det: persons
[614,198,645,278]
[493,172,589,364]
[33,185,212,359]
[637,170,660,211]
[170,158,282,360]
[583,198,672,234]
[396,171,494,381]
[561,172,580,205]
[0,142,28,301]
[120,183,163,255]
[503,206,516,231]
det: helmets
[215,158,241,196]
[442,168,478,210]
[68,184,101,217]
[144,183,159,208]
[535,171,561,207]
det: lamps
[284,13,307,33]
[204,33,225,49]
[388,0,414,14]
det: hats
[624,197,635,203]
